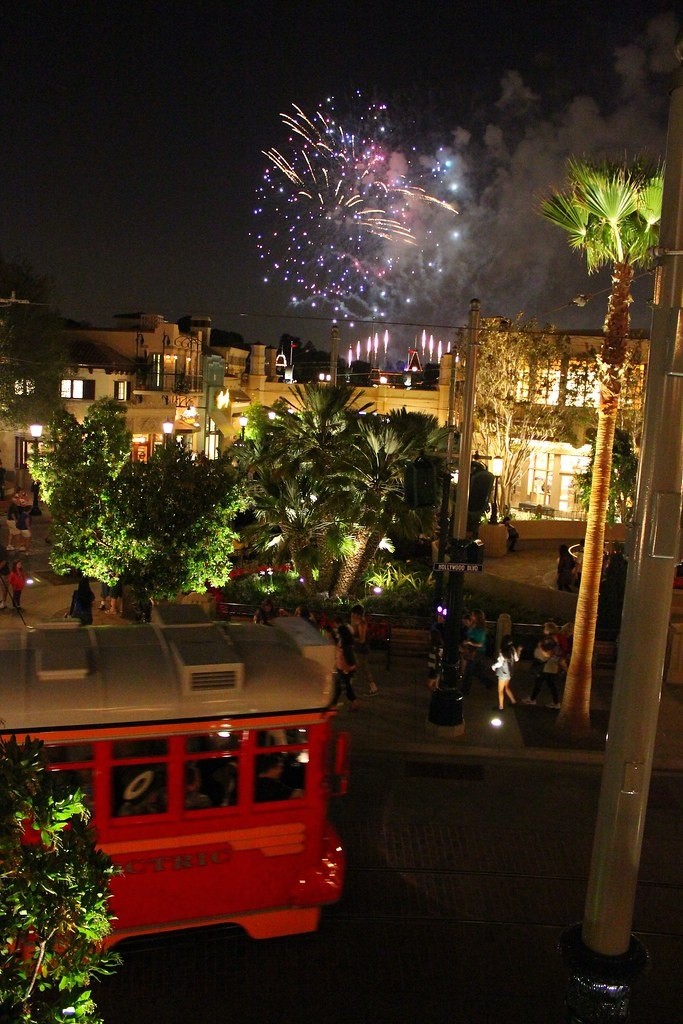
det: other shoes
[508,700,517,708]
[18,606,21,608]
[493,707,504,712]
[347,705,360,712]
[6,546,15,551]
[12,606,15,609]
[328,704,336,710]
[18,547,26,551]
[105,609,117,615]
[363,689,379,696]
[98,605,105,609]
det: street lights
[403,455,495,678]
[28,423,43,516]
[162,416,174,450]
[238,414,247,441]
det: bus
[0,602,351,986]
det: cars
[202,566,295,614]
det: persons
[112,729,295,816]
[69,578,96,625]
[6,503,34,555]
[426,609,575,712]
[0,544,11,608]
[253,597,380,712]
[499,517,519,552]
[8,559,27,607]
[573,539,584,580]
[556,545,575,591]
[98,581,124,615]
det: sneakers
[545,702,560,709]
[521,698,537,704]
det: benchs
[386,625,432,669]
[592,640,618,670]
[228,611,392,671]
